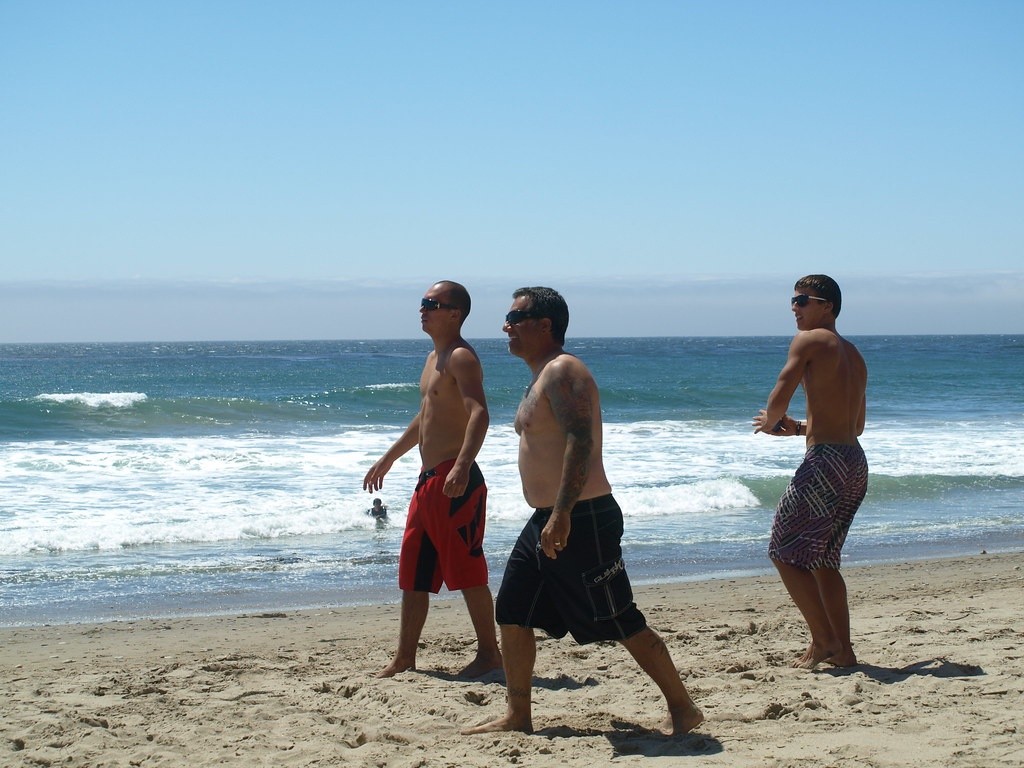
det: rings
[555,542,560,545]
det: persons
[363,280,501,679]
[457,287,705,738]
[367,498,386,519]
[753,275,867,670]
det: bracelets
[796,421,800,435]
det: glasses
[421,298,457,310]
[506,310,551,324]
[792,294,835,307]
